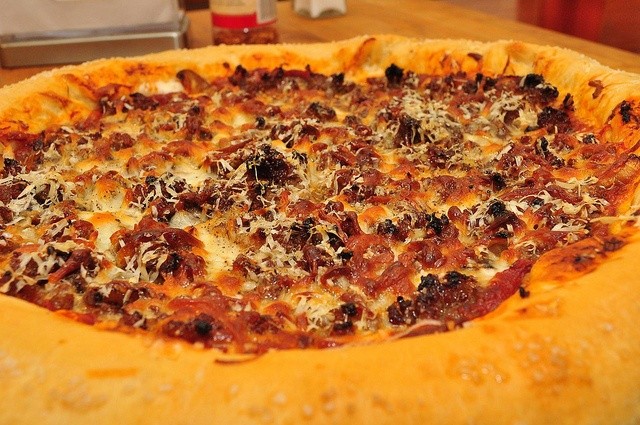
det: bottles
[209,0,280,45]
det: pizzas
[2,36,640,425]
